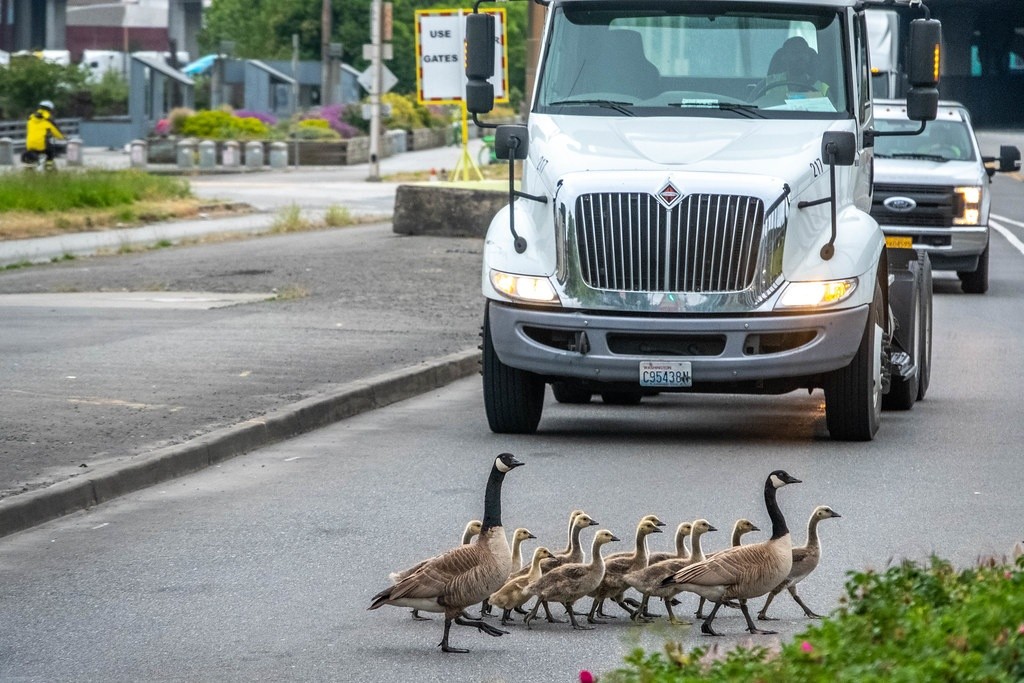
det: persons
[25,99,70,174]
[913,126,966,160]
[745,37,834,104]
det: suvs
[868,97,1022,295]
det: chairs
[767,47,819,76]
[569,30,661,98]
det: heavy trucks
[460,0,942,444]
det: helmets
[39,99,54,111]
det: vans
[782,8,912,101]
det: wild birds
[521,529,621,631]
[622,519,718,625]
[389,520,483,621]
[587,515,667,624]
[757,506,841,621]
[365,453,525,652]
[643,522,693,617]
[478,528,537,617]
[658,469,802,636]
[509,510,589,630]
[488,547,557,627]
